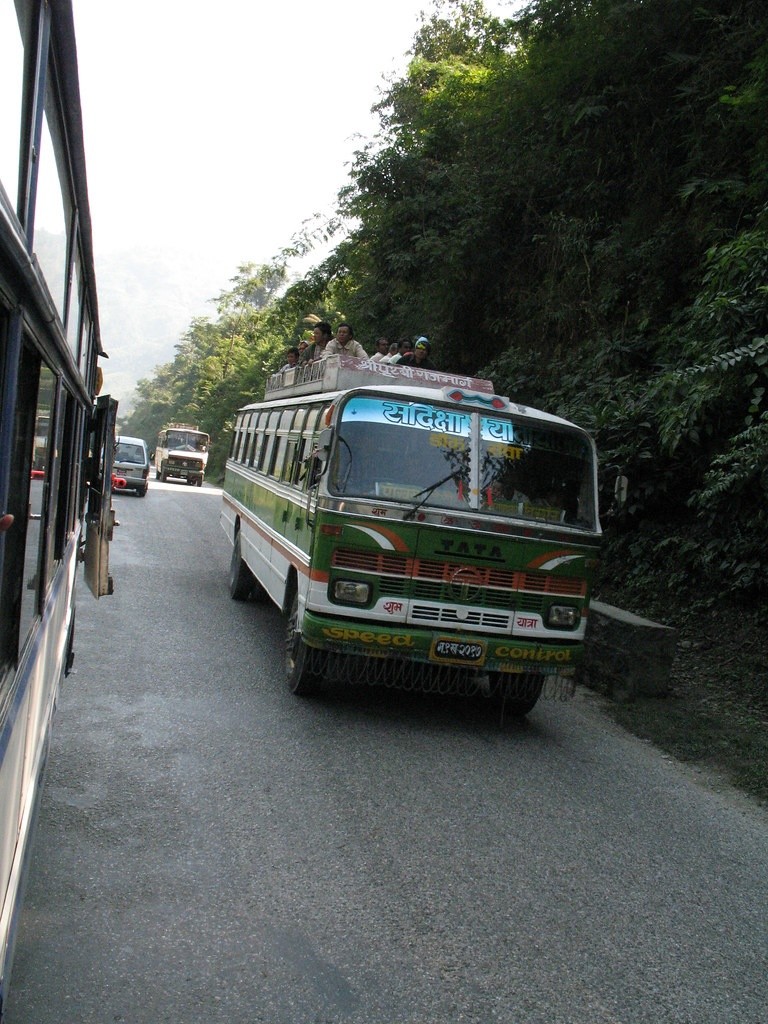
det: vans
[97,434,155,497]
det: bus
[155,422,213,487]
[0,0,128,1024]
[219,353,630,717]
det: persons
[491,469,533,508]
[277,322,437,375]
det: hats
[413,336,430,349]
